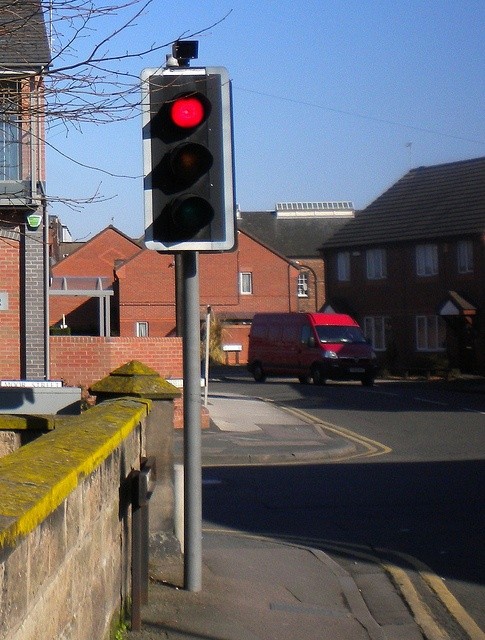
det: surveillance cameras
[172,40,199,60]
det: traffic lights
[139,66,237,254]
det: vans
[247,312,377,387]
